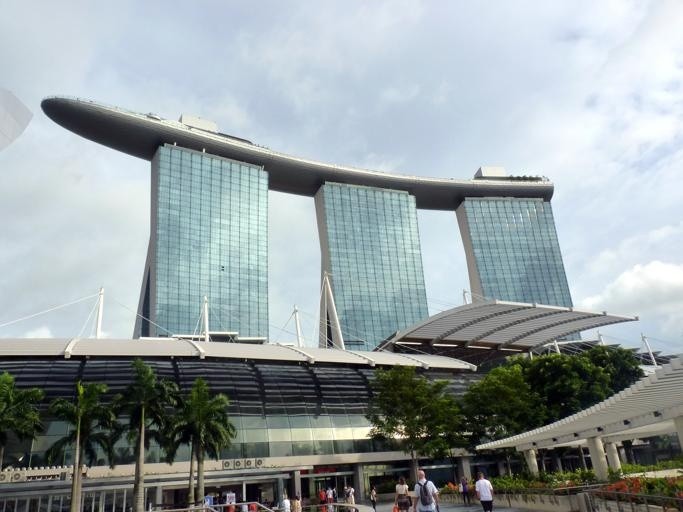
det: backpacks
[417,480,432,505]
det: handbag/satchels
[393,505,398,512]
[407,496,412,505]
[459,484,463,492]
[371,495,376,501]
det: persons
[412,470,439,512]
[291,495,302,512]
[395,476,410,512]
[475,472,494,512]
[370,484,378,512]
[279,494,292,512]
[260,498,268,509]
[319,485,356,504]
[459,476,470,506]
[320,505,356,512]
